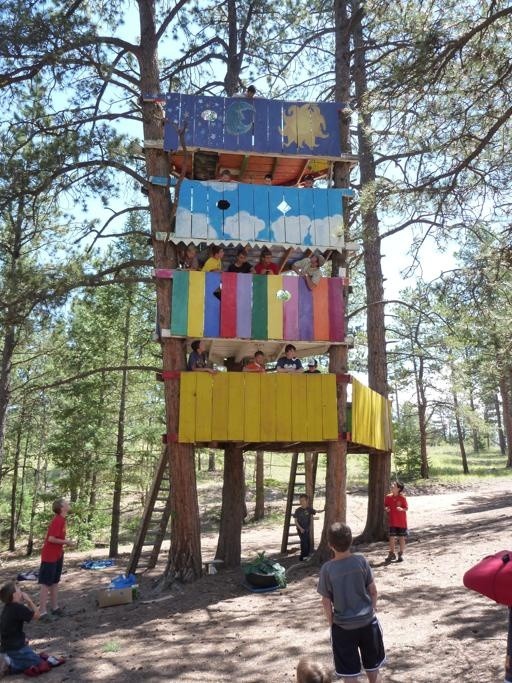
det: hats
[307,358,317,367]
[318,254,325,267]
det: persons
[382,480,408,561]
[297,653,332,683]
[263,173,273,186]
[177,240,327,292]
[1,583,42,676]
[303,174,316,188]
[36,498,76,623]
[317,521,386,683]
[205,169,244,183]
[184,337,324,375]
[293,493,326,561]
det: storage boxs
[98,588,133,608]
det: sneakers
[0,654,11,680]
[385,551,396,562]
[398,552,403,561]
[37,612,52,623]
[51,608,64,616]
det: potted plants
[243,551,287,589]
